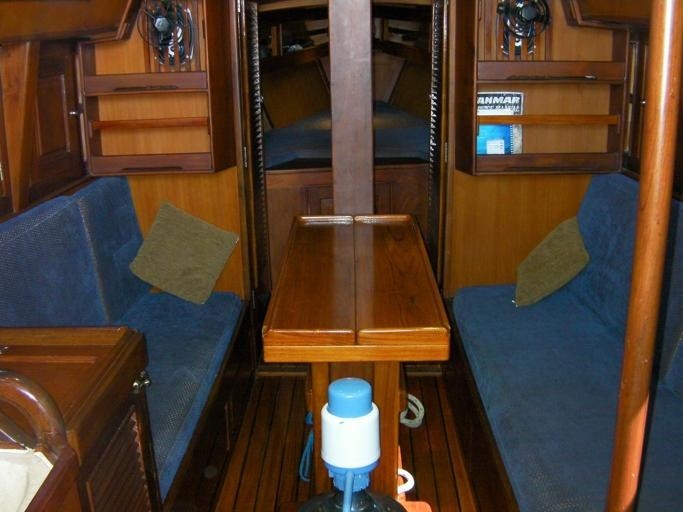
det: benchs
[452,168,683,511]
[0,172,252,511]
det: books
[476,91,525,155]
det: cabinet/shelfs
[454,1,629,177]
[0,43,86,196]
[71,0,236,178]
[0,324,164,511]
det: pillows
[127,198,239,304]
[514,215,590,307]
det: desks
[259,211,451,496]
